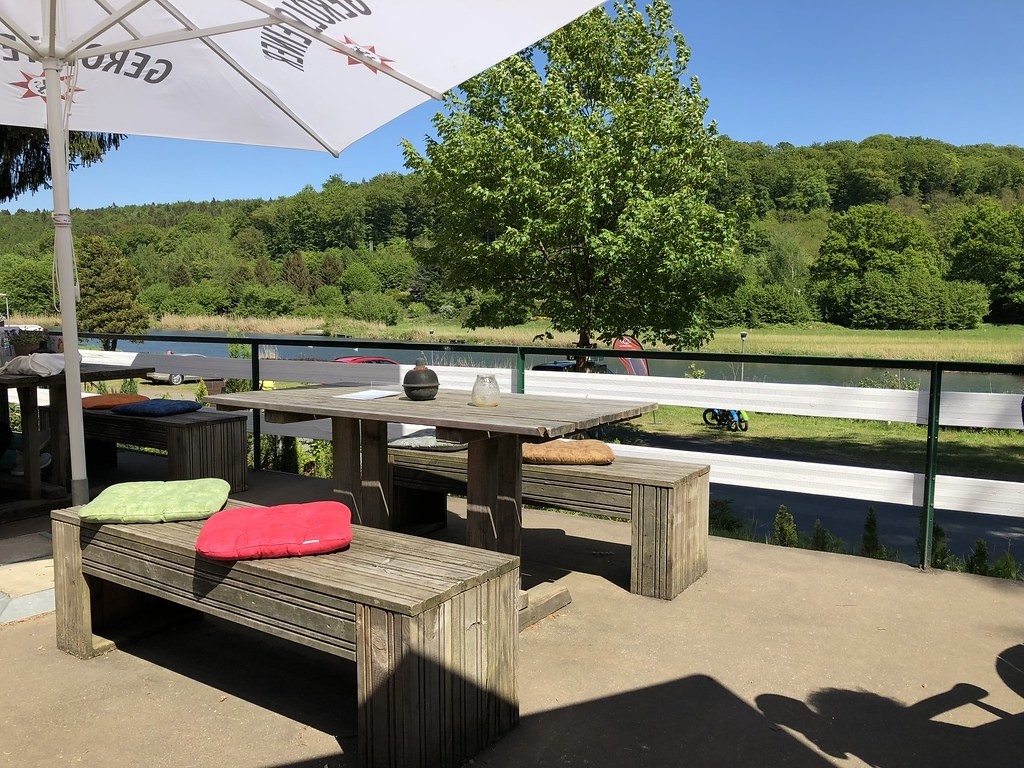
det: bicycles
[703,408,750,432]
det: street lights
[740,332,748,381]
[0,294,10,327]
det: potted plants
[8,327,50,356]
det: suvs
[332,355,400,365]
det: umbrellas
[0,0,608,506]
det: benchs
[38,405,249,496]
[386,427,711,600]
[50,497,521,768]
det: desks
[0,363,155,513]
[203,382,659,634]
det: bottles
[471,374,500,407]
[402,358,441,401]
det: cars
[0,312,4,327]
[532,360,614,374]
[141,353,225,386]
